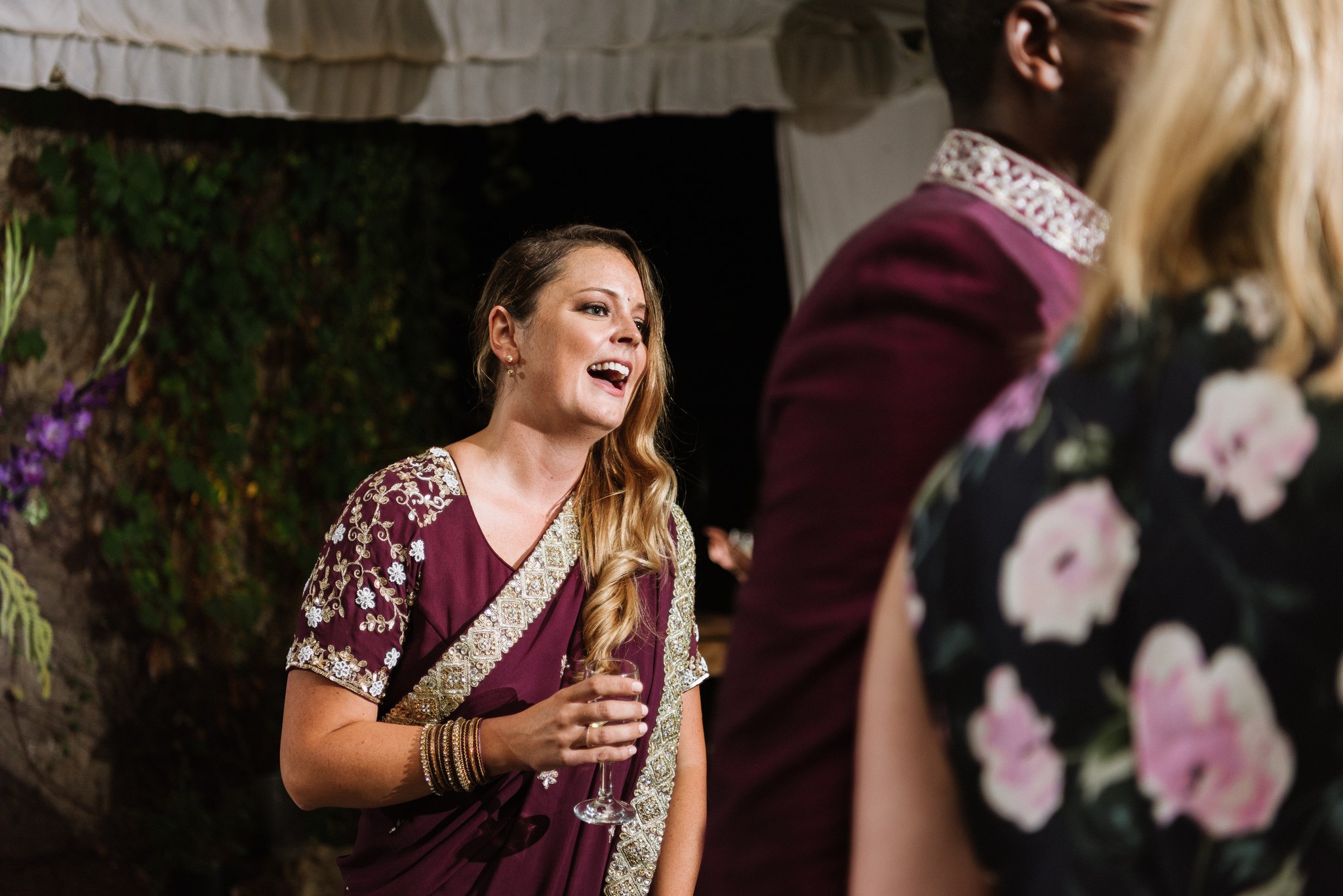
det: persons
[850,2,1343,896]
[704,0,1167,896]
[278,225,711,896]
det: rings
[586,724,592,747]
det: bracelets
[419,717,488,796]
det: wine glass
[574,658,641,825]
